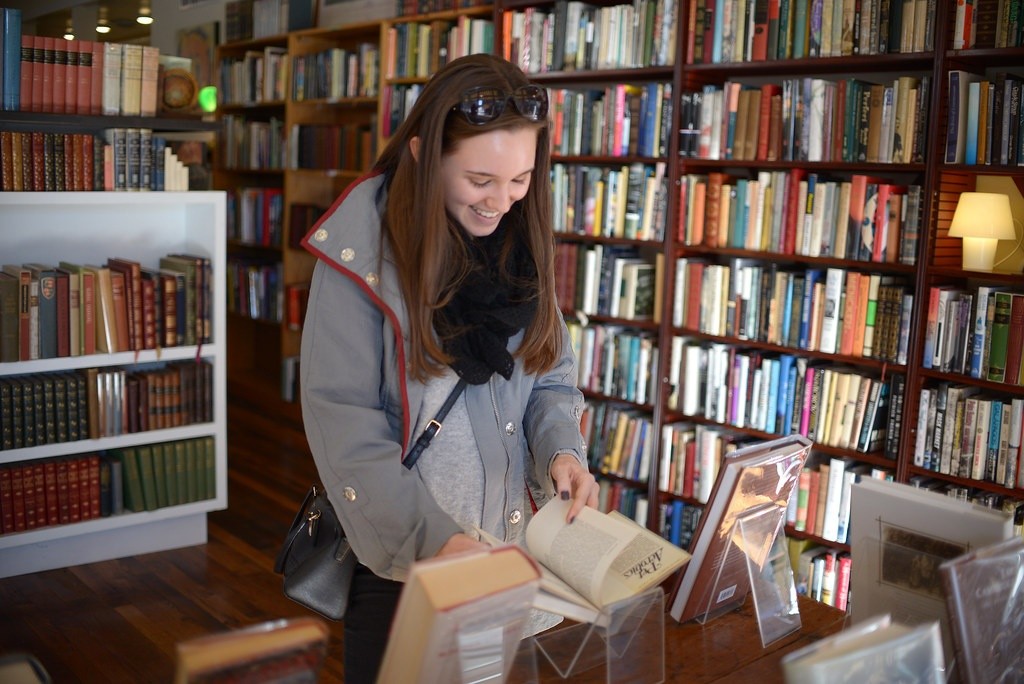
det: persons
[0,0,1024,684]
[301,52,598,684]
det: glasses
[445,85,549,127]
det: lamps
[95,5,113,34]
[62,16,75,42]
[137,0,155,25]
[946,191,1016,273]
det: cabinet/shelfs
[0,108,229,576]
[215,0,1024,606]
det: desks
[507,577,852,684]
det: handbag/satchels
[274,484,357,624]
